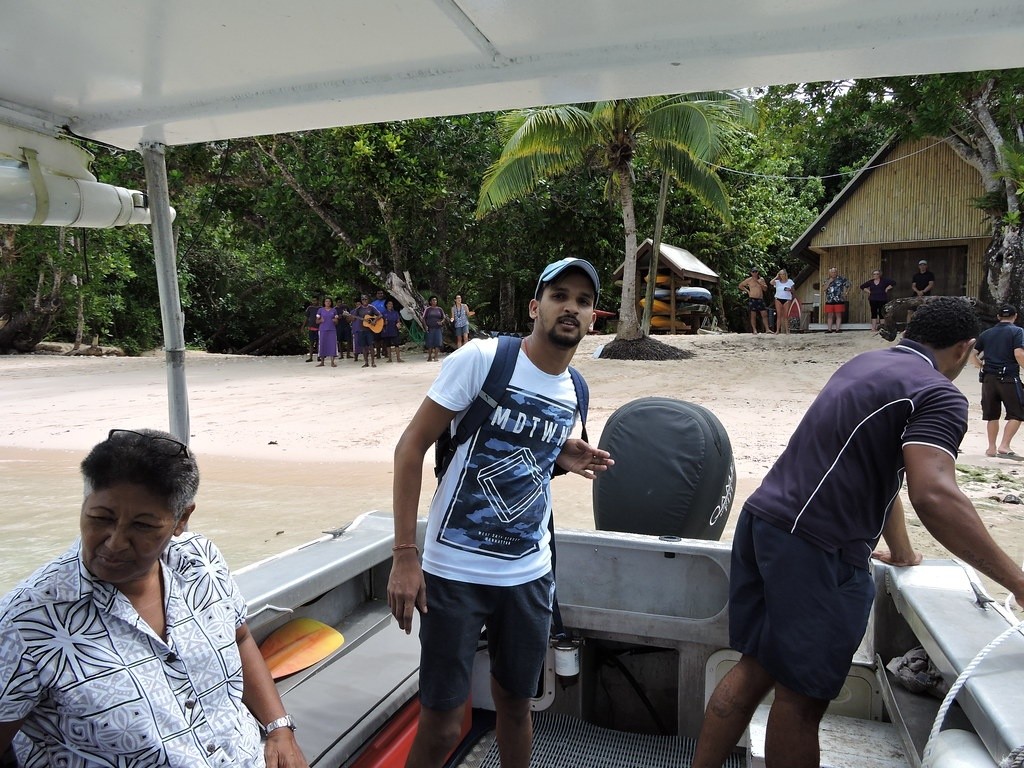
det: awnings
[0,0,1024,450]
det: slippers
[997,450,1024,461]
[986,448,997,456]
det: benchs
[281,609,422,768]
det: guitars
[343,310,384,333]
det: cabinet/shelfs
[649,279,691,335]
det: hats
[353,296,363,306]
[428,295,439,306]
[997,304,1017,318]
[919,260,927,265]
[535,256,600,311]
[751,267,758,272]
[384,299,395,309]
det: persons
[302,296,323,362]
[315,297,338,367]
[450,294,475,348]
[0,427,307,768]
[907,260,935,323]
[334,297,363,359]
[380,299,405,363]
[387,257,615,768]
[860,270,896,330]
[349,289,387,368]
[739,267,774,334]
[970,305,1023,460]
[770,269,795,335]
[691,296,1024,767]
[422,295,446,362]
[821,268,851,333]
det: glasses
[361,299,368,301]
[873,273,879,275]
[107,428,191,459]
[751,271,758,273]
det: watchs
[266,714,296,737]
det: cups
[554,640,580,677]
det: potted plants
[788,317,804,334]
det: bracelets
[922,290,924,293]
[392,543,419,556]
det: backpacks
[434,336,588,486]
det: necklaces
[456,304,461,318]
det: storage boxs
[746,705,912,768]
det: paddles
[259,618,344,679]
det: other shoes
[825,329,832,333]
[766,330,774,334]
[305,351,444,368]
[871,328,876,332]
[835,329,842,333]
[753,331,758,335]
